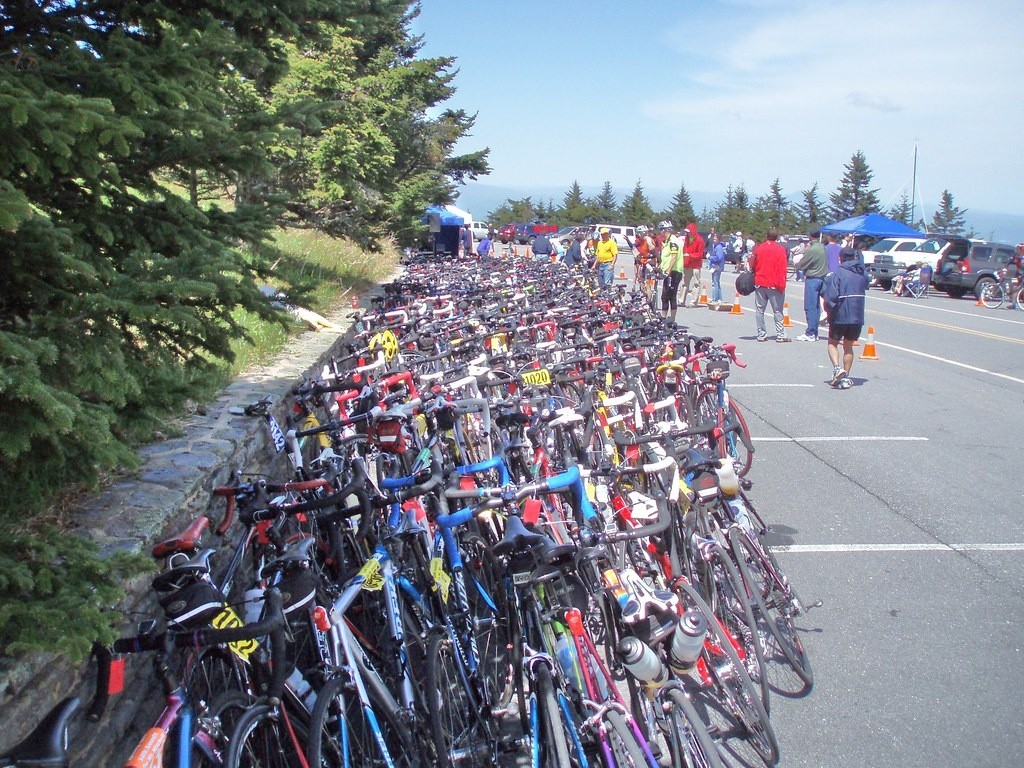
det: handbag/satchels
[734,271,754,295]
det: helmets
[368,329,399,363]
[634,226,649,234]
[657,221,673,229]
[1014,243,1024,255]
[736,232,742,236]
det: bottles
[617,636,661,680]
[728,498,759,555]
[346,493,361,521]
[555,635,590,698]
[284,665,318,713]
[590,653,608,702]
[244,586,266,641]
[593,483,613,524]
[670,611,707,674]
[715,458,739,495]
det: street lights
[525,246,530,257]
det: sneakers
[797,333,819,342]
[831,366,846,386]
[840,377,854,389]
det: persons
[531,226,618,287]
[706,227,756,274]
[1005,243,1024,309]
[460,222,495,257]
[797,229,828,342]
[776,231,864,326]
[749,228,792,342]
[823,247,870,388]
[888,261,929,296]
[706,234,728,305]
[622,220,705,320]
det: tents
[421,205,474,258]
[819,213,927,249]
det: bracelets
[611,264,614,267]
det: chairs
[894,266,931,298]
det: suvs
[514,223,547,246]
[857,237,928,287]
[496,223,520,245]
[471,221,497,243]
[870,232,990,294]
[548,226,588,249]
[933,233,1024,302]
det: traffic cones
[513,246,518,255]
[975,292,988,308]
[551,250,557,263]
[858,324,880,360]
[727,292,747,314]
[501,249,507,259]
[781,302,794,328]
[649,277,653,286]
[618,264,627,280]
[697,284,710,304]
[489,248,495,257]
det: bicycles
[0,232,824,768]
[786,250,797,280]
[979,267,1024,313]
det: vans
[588,223,638,252]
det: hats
[684,228,689,233]
[828,231,840,240]
[600,227,610,235]
[810,230,821,238]
[839,248,854,263]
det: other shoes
[818,317,829,328]
[794,279,802,282]
[756,336,767,342]
[686,301,698,309]
[893,291,901,297]
[1006,305,1016,310]
[732,271,740,274]
[776,336,793,343]
[677,300,685,307]
